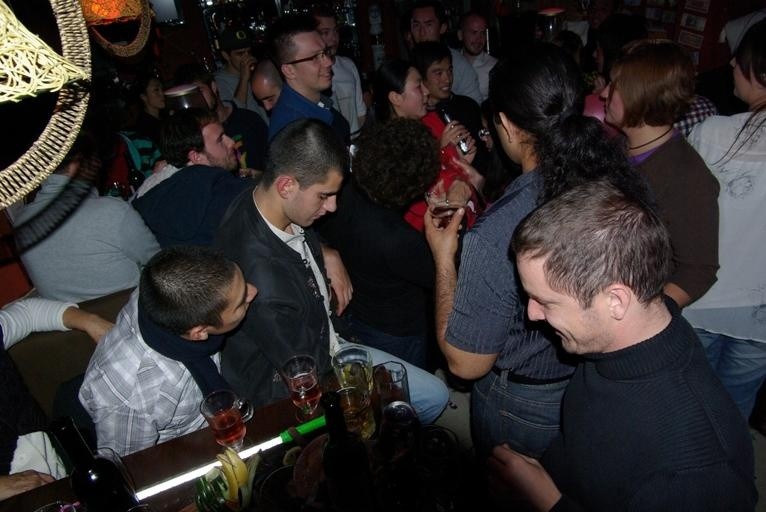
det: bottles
[440,106,469,154]
[49,414,141,512]
[319,391,367,488]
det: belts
[491,369,577,388]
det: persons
[135,107,237,201]
[214,26,270,125]
[215,117,354,405]
[263,14,351,156]
[456,10,499,100]
[252,59,284,111]
[78,245,258,458]
[424,47,646,460]
[177,67,267,171]
[684,21,766,418]
[407,2,483,106]
[313,9,367,140]
[335,118,441,318]
[600,45,720,310]
[409,41,492,176]
[0,297,115,500]
[355,60,476,234]
[133,74,164,135]
[492,176,756,511]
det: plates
[293,434,330,503]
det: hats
[213,27,257,49]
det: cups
[338,387,365,432]
[373,362,411,405]
[331,346,373,399]
[426,189,470,234]
[279,355,322,408]
[92,447,136,493]
[200,389,255,445]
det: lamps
[1,2,90,207]
[79,1,151,56]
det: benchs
[7,286,146,419]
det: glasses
[284,44,333,65]
